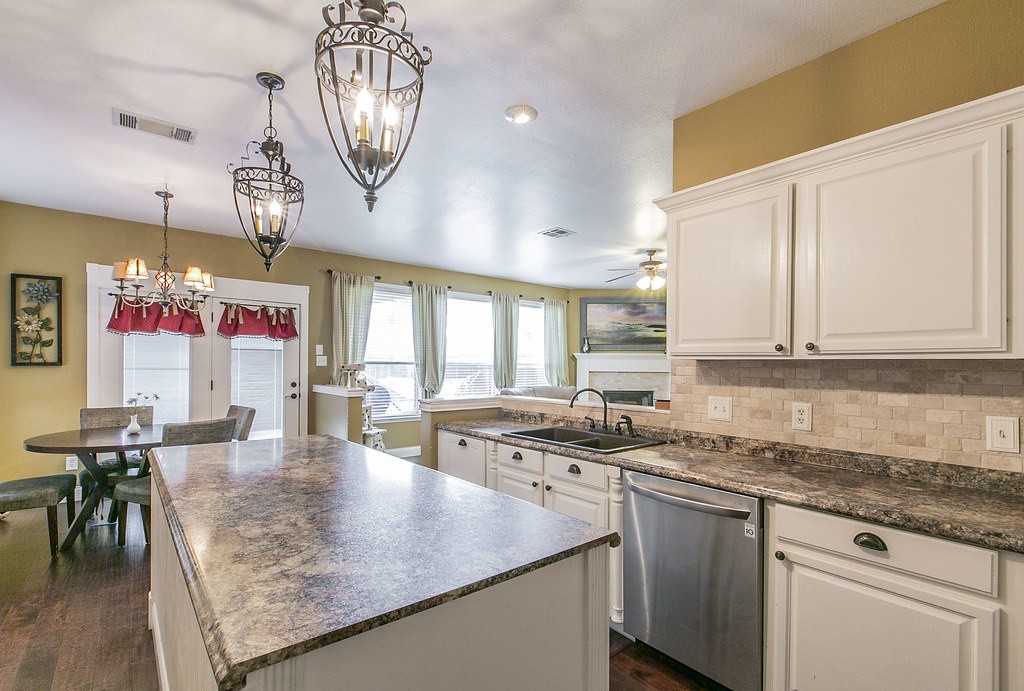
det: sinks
[556,434,668,454]
[501,426,602,444]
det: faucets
[568,387,608,433]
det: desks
[145,433,621,691]
[22,423,167,551]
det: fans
[605,250,667,284]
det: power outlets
[791,402,813,432]
[65,457,79,470]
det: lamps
[635,267,666,291]
[226,72,305,271]
[108,191,214,317]
[314,0,434,214]
[505,105,538,125]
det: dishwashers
[619,468,766,691]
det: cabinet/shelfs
[652,90,1010,361]
[764,503,1024,691]
[438,430,487,489]
[494,443,609,532]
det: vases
[582,337,591,353]
[126,414,141,433]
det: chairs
[0,405,257,556]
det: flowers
[127,392,161,416]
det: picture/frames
[578,296,666,351]
[10,273,63,366]
